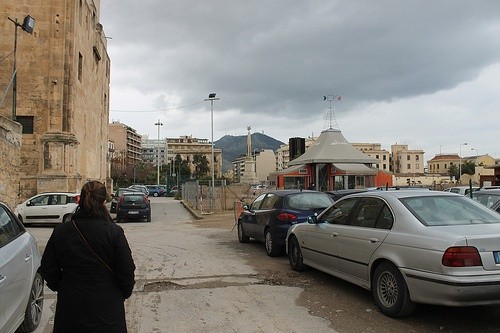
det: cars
[253,185,266,194]
[0,202,45,333]
[109,184,182,223]
[14,191,80,227]
[236,186,500,318]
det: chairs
[52,197,61,205]
[359,206,388,229]
[267,196,278,208]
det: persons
[308,183,315,190]
[41,181,136,333]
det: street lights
[204,93,221,211]
[459,142,468,184]
[154,119,164,185]
[470,147,478,167]
[7,15,36,121]
